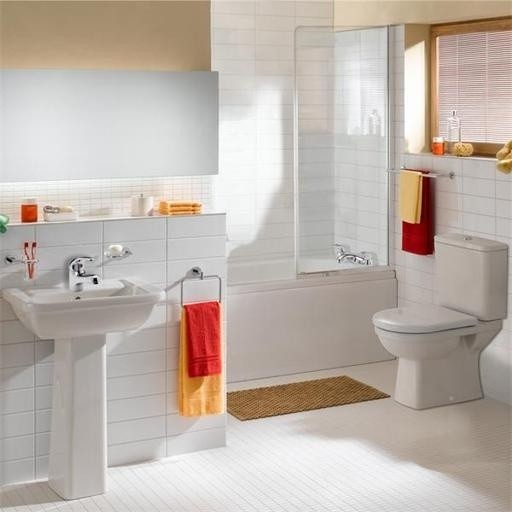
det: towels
[178,302,225,418]
[397,167,437,258]
[159,199,204,216]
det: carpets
[226,373,392,423]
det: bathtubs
[228,254,399,385]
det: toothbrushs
[31,241,37,276]
[24,241,31,276]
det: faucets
[67,256,100,292]
[337,252,360,264]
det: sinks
[3,281,166,342]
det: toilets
[372,235,507,409]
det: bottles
[20,196,38,224]
[431,109,462,155]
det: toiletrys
[19,197,38,222]
[430,137,445,156]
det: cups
[132,195,155,217]
